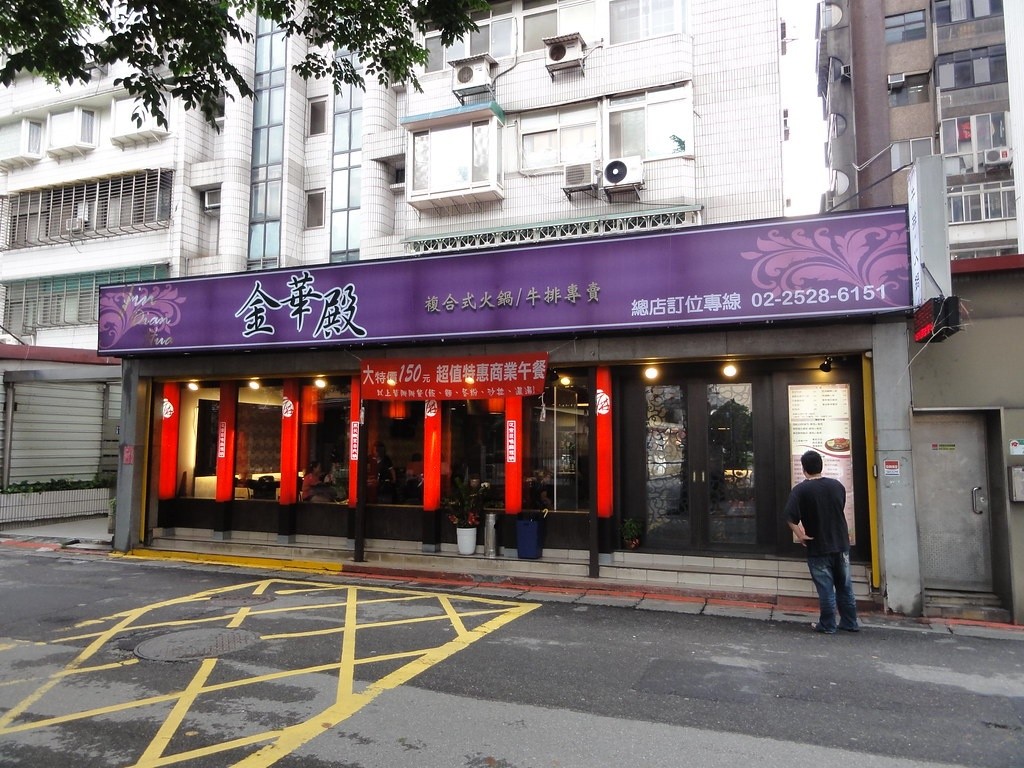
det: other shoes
[811,623,833,634]
[838,624,860,632]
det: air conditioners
[545,38,583,73]
[601,156,647,194]
[563,158,599,191]
[66,218,83,231]
[204,187,221,211]
[453,59,492,94]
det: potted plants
[443,462,495,557]
[618,514,644,550]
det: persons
[302,460,332,504]
[368,442,400,504]
[782,450,862,633]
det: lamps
[818,356,833,372]
